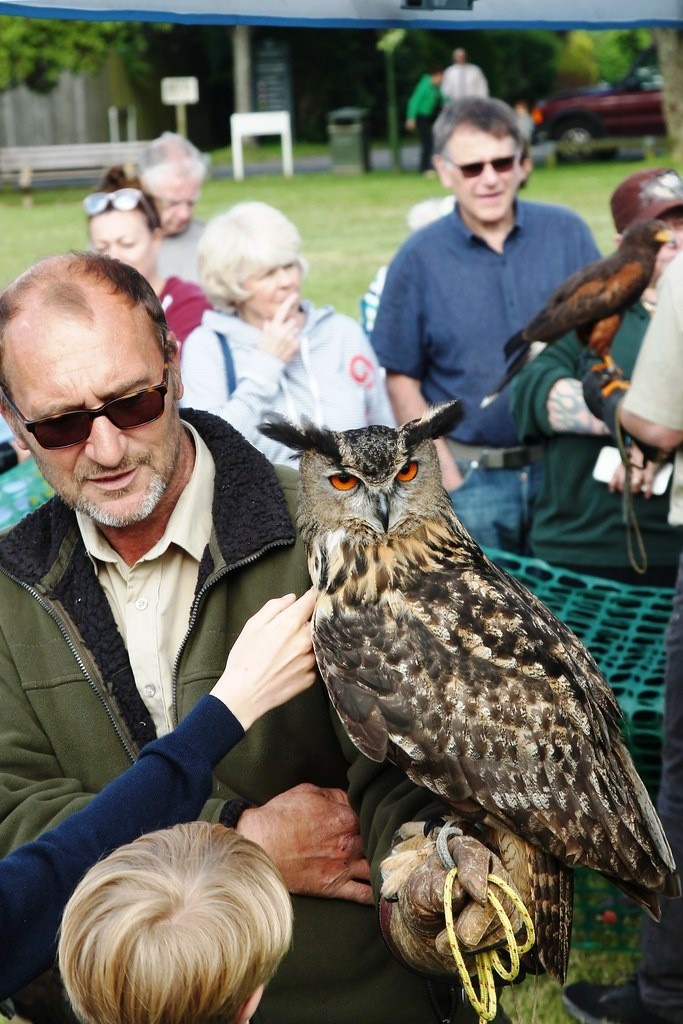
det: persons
[1,588,318,1005]
[181,205,400,472]
[57,822,293,1024]
[511,169,683,680]
[371,97,604,576]
[408,68,453,177]
[86,168,211,346]
[137,132,209,290]
[0,250,459,1024]
[442,49,491,101]
[560,251,683,1024]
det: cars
[530,65,666,161]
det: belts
[446,436,545,470]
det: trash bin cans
[326,107,371,179]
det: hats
[611,169,683,238]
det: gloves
[382,819,534,978]
[580,359,631,443]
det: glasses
[0,329,170,450]
[439,142,522,178]
[82,186,159,230]
